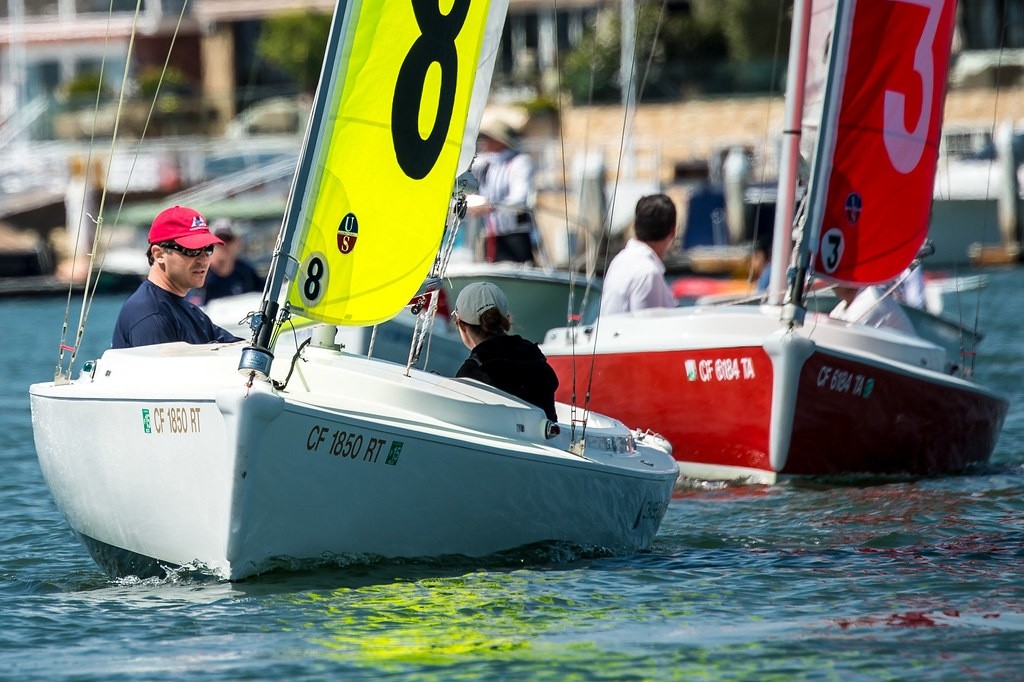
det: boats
[197,253,613,375]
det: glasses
[159,243,214,258]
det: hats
[148,206,225,249]
[451,281,510,326]
[480,119,521,151]
[211,219,235,236]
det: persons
[181,217,271,308]
[598,192,679,316]
[464,116,537,266]
[829,284,919,337]
[450,280,560,422]
[109,205,245,348]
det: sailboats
[527,0,1014,480]
[27,0,686,585]
